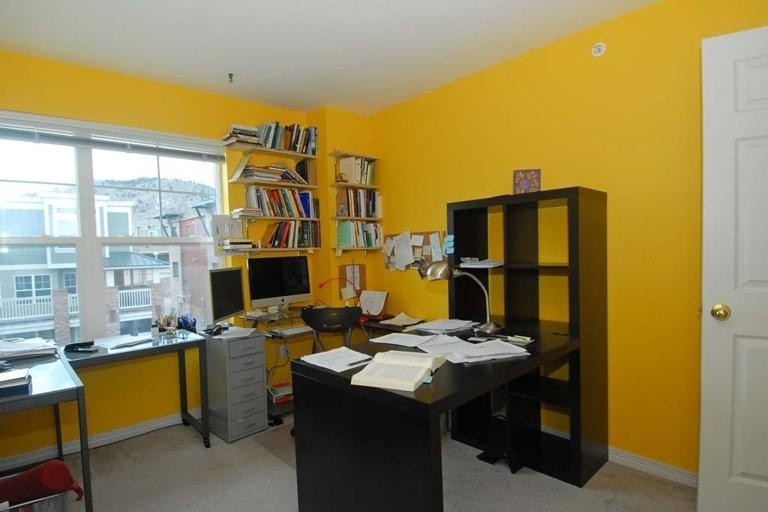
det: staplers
[64,341,99,353]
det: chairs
[298,305,371,358]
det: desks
[289,318,579,510]
[1,324,210,512]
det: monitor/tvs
[248,256,310,316]
[209,266,245,323]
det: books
[222,121,321,250]
[335,156,383,249]
[350,349,448,392]
[270,384,294,399]
[0,368,33,398]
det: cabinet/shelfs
[447,185,611,488]
[199,327,269,444]
[229,143,382,250]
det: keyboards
[280,325,314,335]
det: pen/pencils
[349,358,372,366]
[159,314,197,329]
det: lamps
[427,261,507,335]
